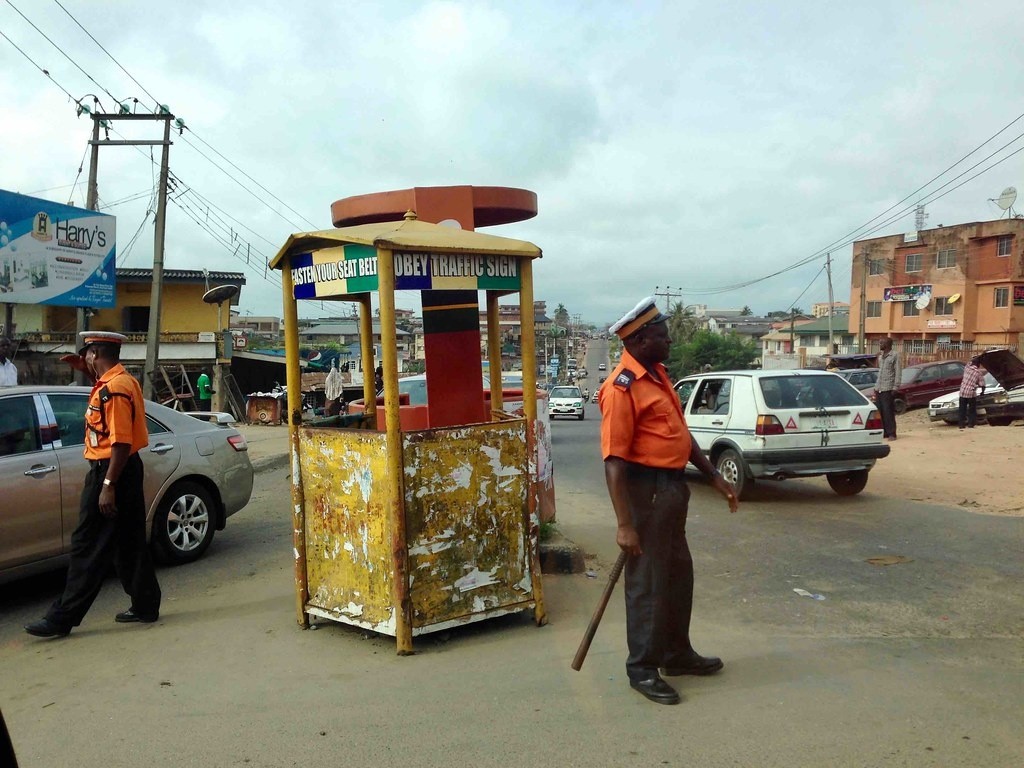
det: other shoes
[888,436,896,441]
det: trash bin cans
[245,393,284,427]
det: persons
[597,296,739,704]
[0,335,18,387]
[873,337,902,441]
[23,331,161,637]
[958,355,985,431]
[197,367,217,421]
[582,388,589,397]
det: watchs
[103,478,116,486]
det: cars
[927,371,1005,425]
[374,375,491,406]
[599,375,608,383]
[837,368,880,398]
[592,391,600,404]
[548,386,585,421]
[598,364,606,371]
[859,360,967,415]
[673,368,890,497]
[0,384,255,567]
[568,358,587,379]
[973,349,1024,427]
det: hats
[609,295,673,339]
[79,330,128,356]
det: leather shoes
[660,649,724,677]
[629,676,681,705]
[24,618,72,637]
[115,610,158,624]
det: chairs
[555,392,564,398]
[700,394,717,413]
[64,417,88,446]
